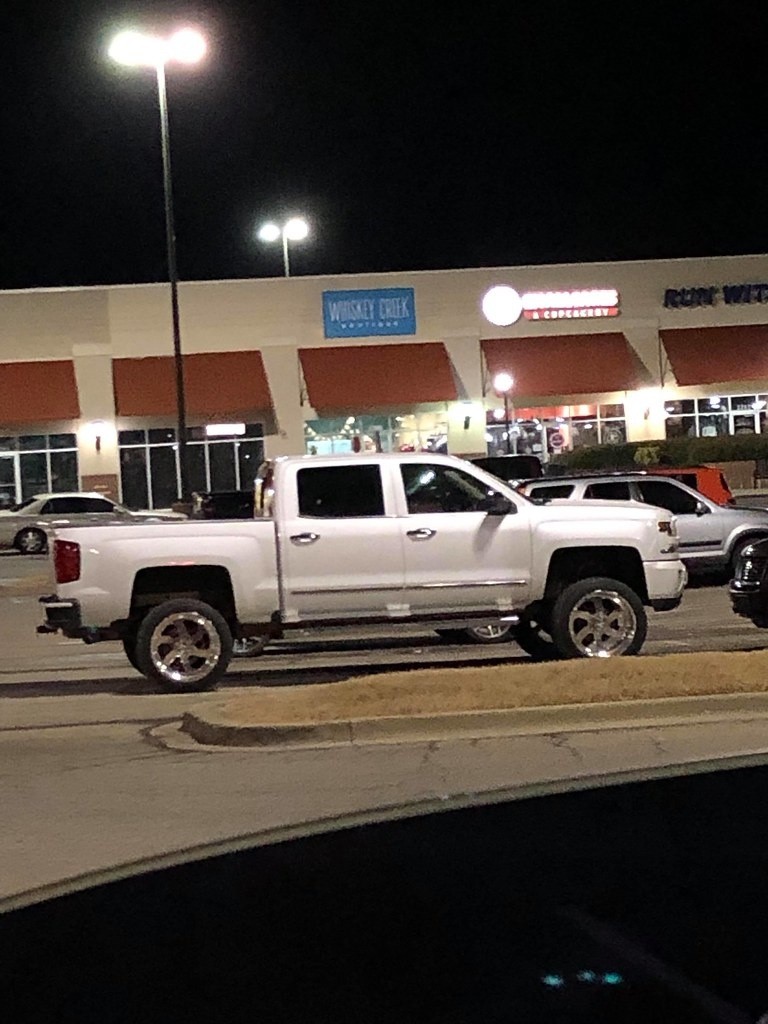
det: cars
[727,536,768,631]
[518,473,768,575]
[0,490,189,553]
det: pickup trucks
[36,449,689,693]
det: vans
[410,454,544,506]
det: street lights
[112,28,200,504]
[260,219,303,282]
[495,373,514,455]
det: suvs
[500,466,738,510]
